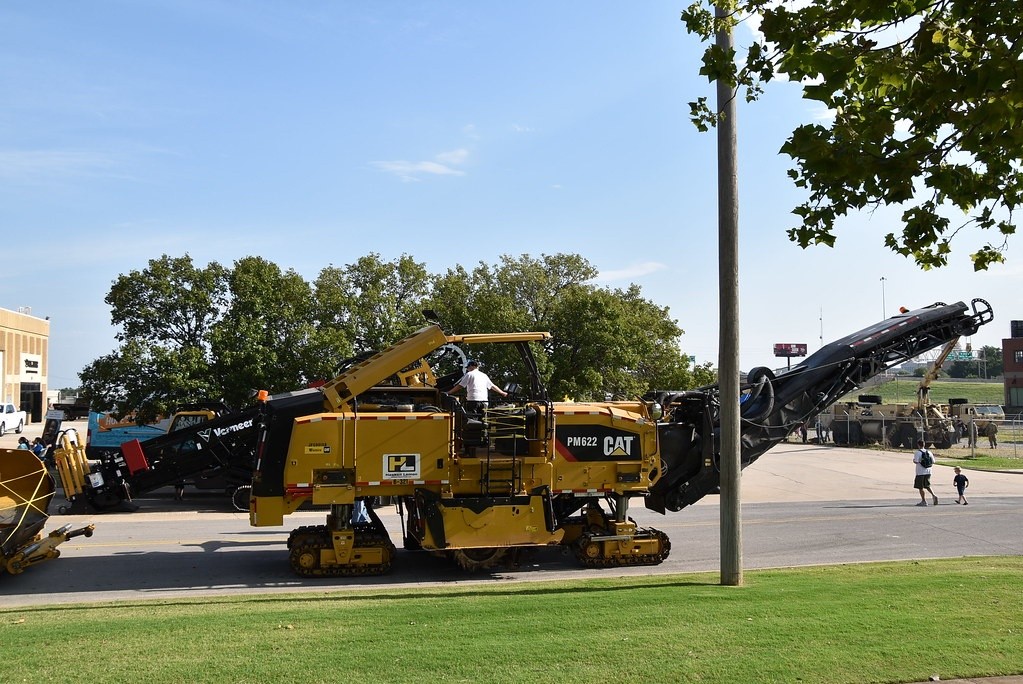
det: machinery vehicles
[0,448,95,575]
[52,298,1005,577]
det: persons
[984,421,998,449]
[351,499,369,523]
[816,423,826,443]
[32,436,47,461]
[952,466,969,505]
[912,439,938,506]
[967,418,979,448]
[18,437,30,450]
[446,362,507,447]
[800,425,808,443]
[824,428,831,442]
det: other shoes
[955,499,961,504]
[917,500,928,506]
[933,496,938,507]
[965,502,968,505]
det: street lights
[878,277,888,378]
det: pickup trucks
[0,403,27,437]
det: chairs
[30,443,51,460]
[440,391,488,430]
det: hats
[465,361,478,369]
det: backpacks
[920,449,932,468]
